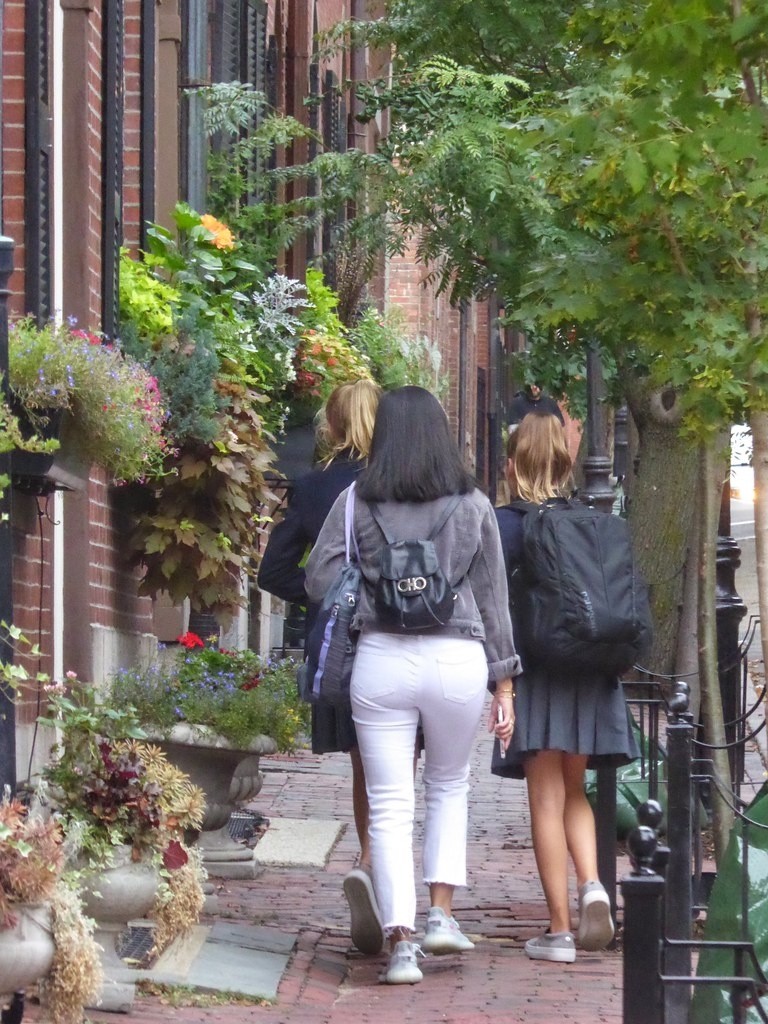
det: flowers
[6,307,181,488]
[291,329,374,408]
[105,620,316,833]
[140,198,257,283]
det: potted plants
[31,732,208,962]
[0,781,105,1024]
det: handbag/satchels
[299,480,364,704]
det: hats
[535,381,543,391]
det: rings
[510,721,513,725]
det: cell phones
[497,704,506,758]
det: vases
[9,391,95,497]
[118,715,277,863]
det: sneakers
[577,880,615,951]
[525,926,576,963]
[343,869,385,955]
[422,906,475,956]
[385,942,423,984]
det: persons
[491,414,643,963]
[508,387,566,432]
[305,385,524,984]
[287,381,424,955]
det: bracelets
[495,690,515,698]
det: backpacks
[496,488,654,693]
[365,492,475,629]
